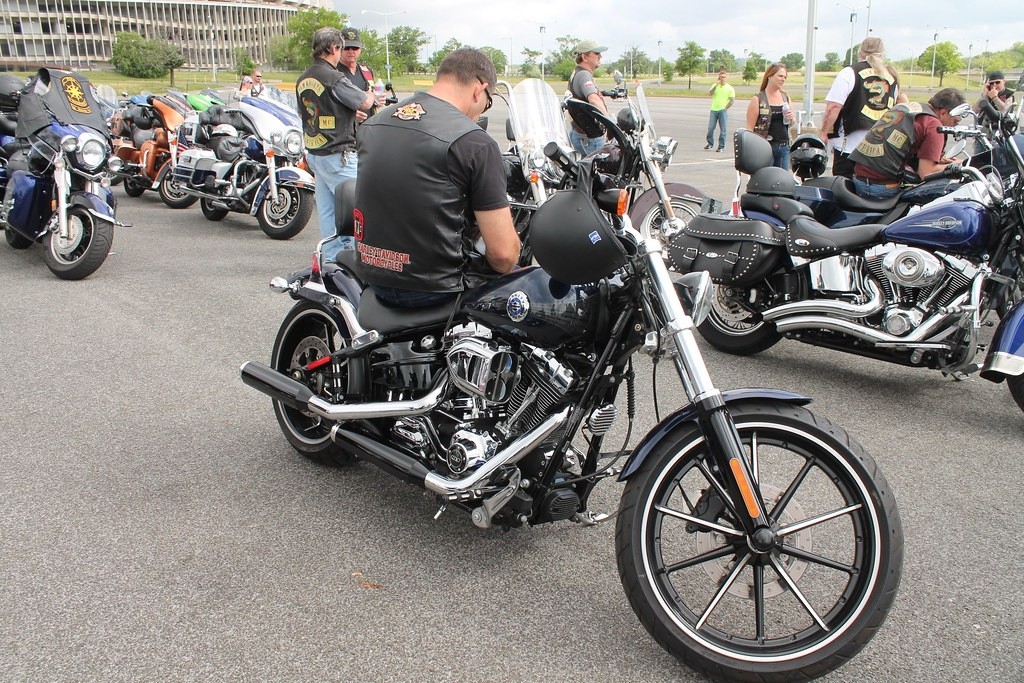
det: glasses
[256,75,262,77]
[341,46,359,51]
[476,74,493,114]
[989,81,1003,85]
[248,82,254,84]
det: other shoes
[704,145,713,149]
[716,148,723,152]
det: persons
[704,72,736,153]
[972,71,1015,157]
[848,88,968,185]
[294,25,375,263]
[352,49,523,301]
[746,63,796,173]
[238,75,253,96]
[341,27,386,127]
[249,70,266,96]
[819,37,902,179]
[568,40,611,159]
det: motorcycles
[236,81,906,682]
[0,62,318,282]
[487,99,1024,413]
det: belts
[855,175,900,184]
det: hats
[340,27,362,48]
[990,71,1004,80]
[576,39,608,53]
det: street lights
[926,24,947,88]
[361,9,408,91]
[539,26,547,81]
[836,2,869,65]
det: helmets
[212,124,239,137]
[528,189,629,285]
[0,74,26,111]
[787,133,828,178]
[134,117,155,130]
[616,108,645,133]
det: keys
[342,155,347,165]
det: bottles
[782,102,790,124]
[610,113,617,124]
[373,77,385,105]
